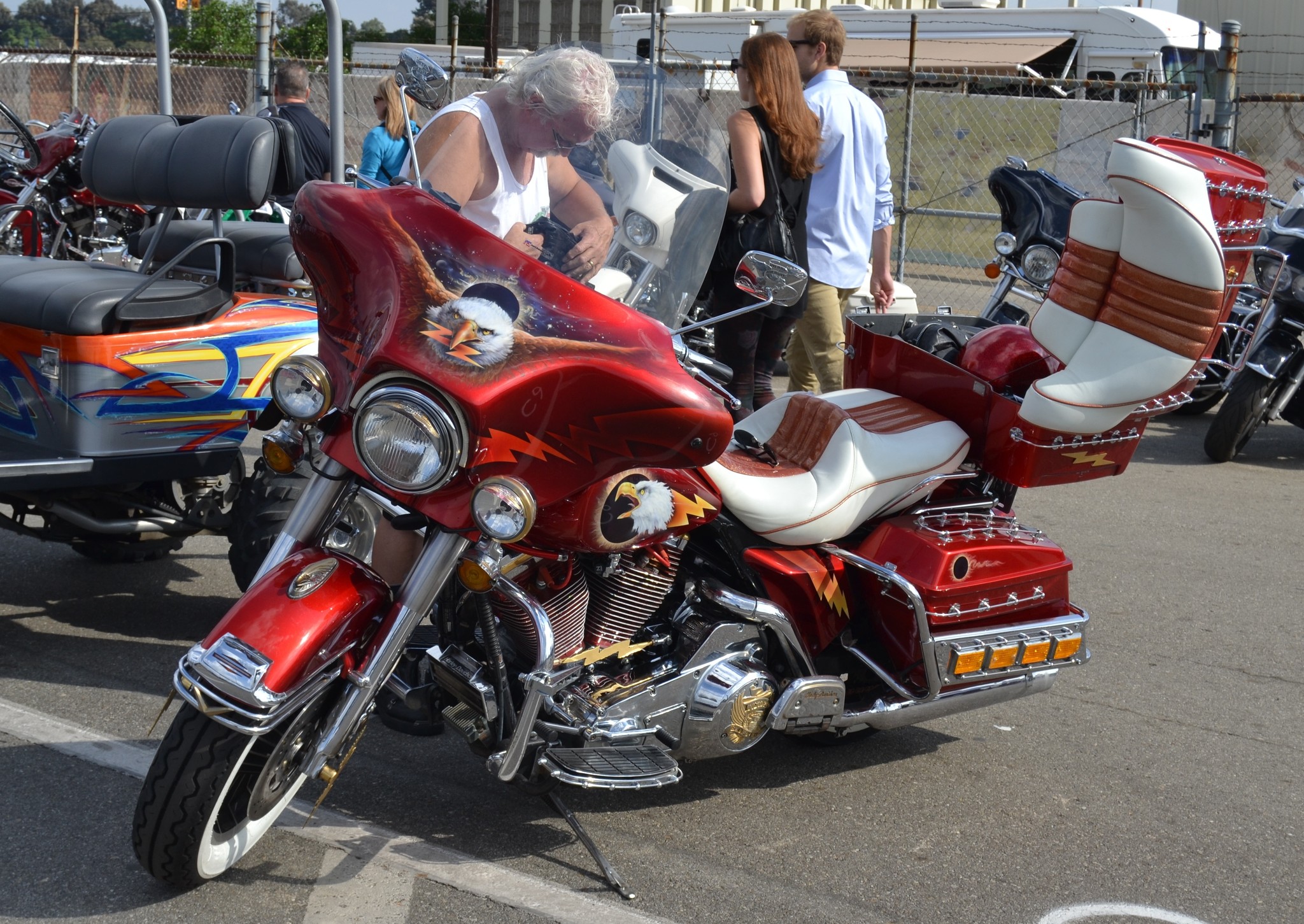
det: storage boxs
[839,131,1275,487]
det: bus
[608,3,1223,106]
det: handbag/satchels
[712,211,798,287]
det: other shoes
[375,691,446,734]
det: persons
[258,48,618,283]
[709,9,896,413]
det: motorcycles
[969,135,1302,459]
[0,53,916,601]
[129,47,1267,907]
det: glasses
[550,120,591,152]
[374,96,384,105]
[789,40,813,48]
[730,59,747,74]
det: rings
[588,260,595,268]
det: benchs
[0,110,308,343]
